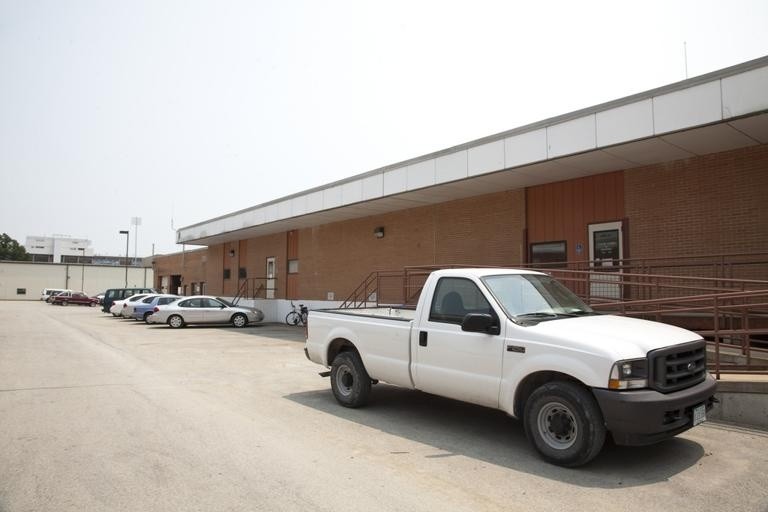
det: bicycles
[286,300,307,326]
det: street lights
[78,248,85,290]
[120,230,129,288]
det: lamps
[372,227,384,241]
[227,250,234,258]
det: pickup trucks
[304,268,718,468]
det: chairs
[439,290,467,317]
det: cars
[41,288,264,328]
